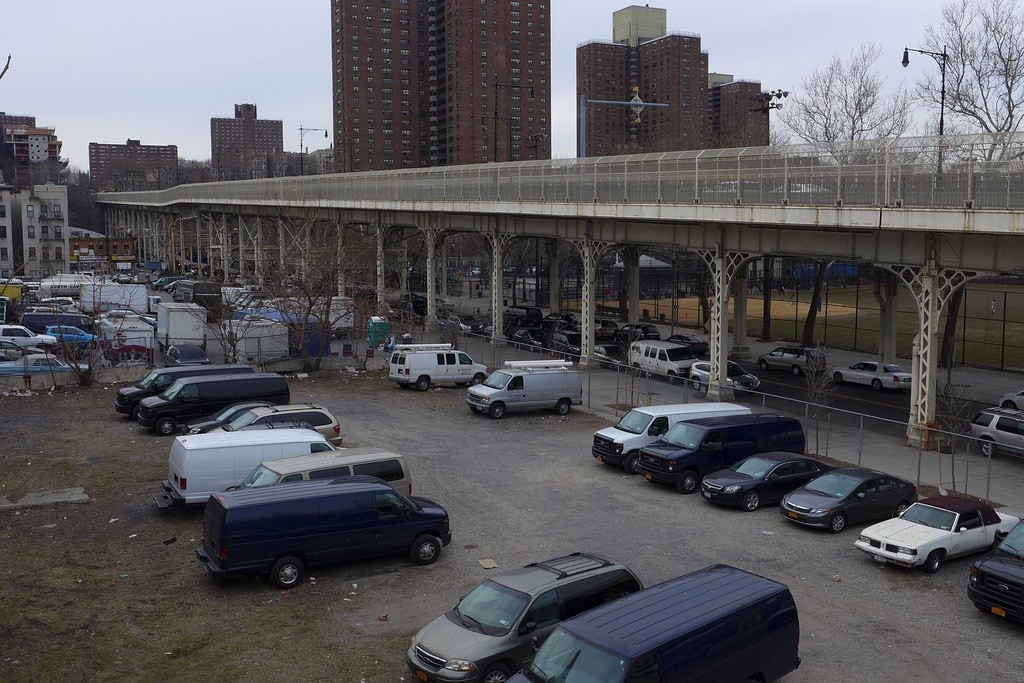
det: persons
[379,336,397,352]
[992,299,997,313]
[604,287,611,301]
[778,281,787,295]
[818,297,823,312]
[790,295,796,306]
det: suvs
[967,407,1024,461]
[203,401,344,446]
[404,551,645,683]
[756,346,828,378]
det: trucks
[154,301,209,352]
[218,313,291,365]
[78,282,165,319]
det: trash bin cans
[401,333,413,344]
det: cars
[179,400,279,438]
[689,360,761,395]
[779,466,919,535]
[342,283,709,385]
[699,450,842,512]
[998,388,1024,412]
[0,257,354,376]
[852,494,1022,574]
[966,517,1023,629]
[830,361,912,393]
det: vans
[387,341,488,392]
[221,447,414,514]
[633,413,806,495]
[591,401,755,476]
[503,561,802,683]
[152,428,349,513]
[463,359,584,420]
[194,474,454,592]
[114,362,256,421]
[136,370,291,438]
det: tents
[608,254,673,268]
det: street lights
[296,124,328,176]
[901,43,950,192]
